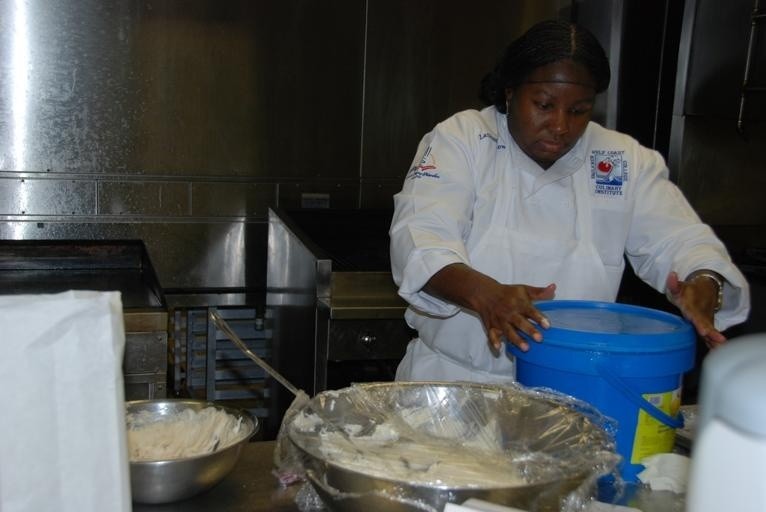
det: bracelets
[690,273,724,310]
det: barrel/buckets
[502,300,698,507]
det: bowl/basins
[285,382,612,512]
[126,396,262,505]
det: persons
[388,18,751,383]
